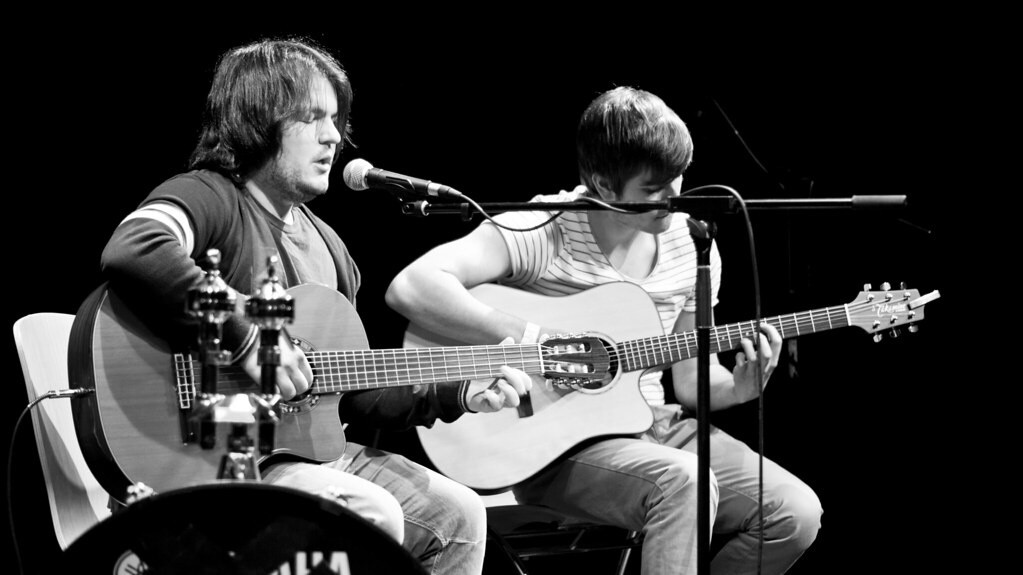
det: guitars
[405,279,939,492]
[69,285,588,497]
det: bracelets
[521,322,541,345]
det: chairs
[482,504,638,575]
[11,313,118,550]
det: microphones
[343,158,463,199]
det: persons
[387,85,825,575]
[101,40,488,575]
